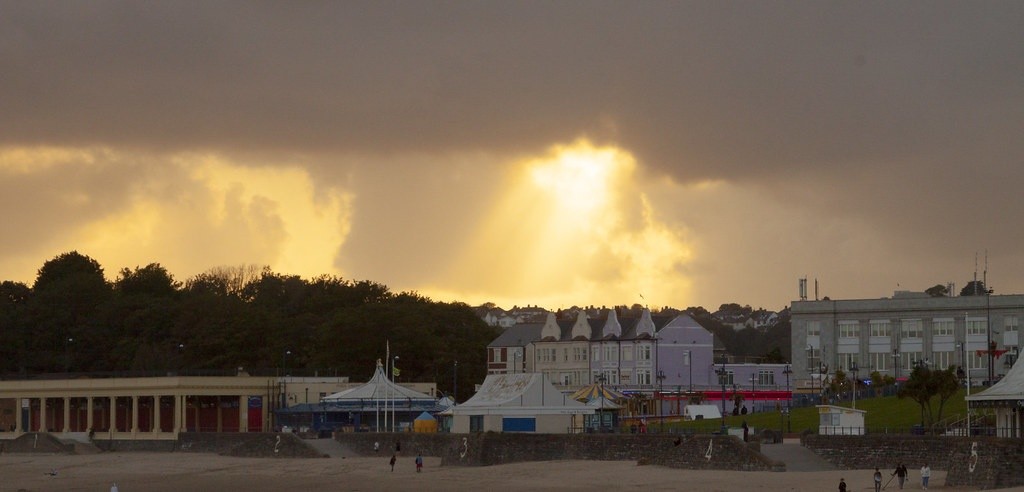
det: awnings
[656,391,793,401]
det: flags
[393,367,400,376]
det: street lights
[782,365,794,433]
[849,362,860,410]
[598,372,606,433]
[956,340,967,369]
[805,344,826,408]
[656,369,667,433]
[719,354,730,434]
[392,355,399,432]
[890,349,902,394]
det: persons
[674,436,681,446]
[374,440,380,457]
[839,478,846,492]
[395,440,401,455]
[893,460,908,490]
[390,455,396,472]
[631,421,647,434]
[415,454,422,472]
[873,467,883,492]
[744,425,749,441]
[921,463,930,490]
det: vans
[684,404,724,421]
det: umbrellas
[567,383,631,427]
[432,396,457,428]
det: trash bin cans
[774,431,782,443]
[696,415,703,419]
[607,428,614,433]
[587,427,594,433]
[970,425,979,435]
[912,425,924,435]
